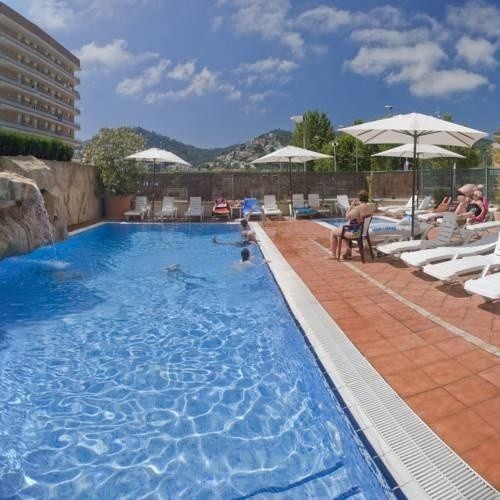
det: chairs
[122,191,354,224]
[370,193,499,303]
[337,214,374,263]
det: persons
[250,144,334,207]
[334,111,489,242]
[232,245,273,267]
[123,147,193,210]
[454,190,485,220]
[213,229,261,248]
[323,188,374,260]
[370,143,468,209]
[238,219,251,238]
